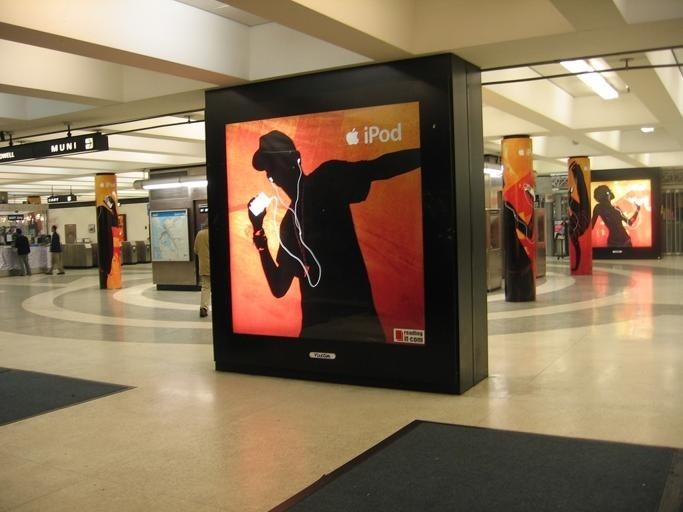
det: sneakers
[45,271,66,276]
[198,308,208,318]
[20,272,31,276]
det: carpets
[272,420,682,512]
[0,364,137,429]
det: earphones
[297,158,301,163]
[606,191,610,195]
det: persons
[15,229,31,276]
[503,177,536,302]
[194,220,213,317]
[97,196,118,288]
[248,130,421,343]
[46,225,65,275]
[569,161,590,271]
[592,185,640,247]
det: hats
[251,128,303,173]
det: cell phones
[250,193,270,215]
[103,197,114,209]
[527,186,536,202]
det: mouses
[269,177,273,182]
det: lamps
[132,172,207,192]
[558,60,619,102]
